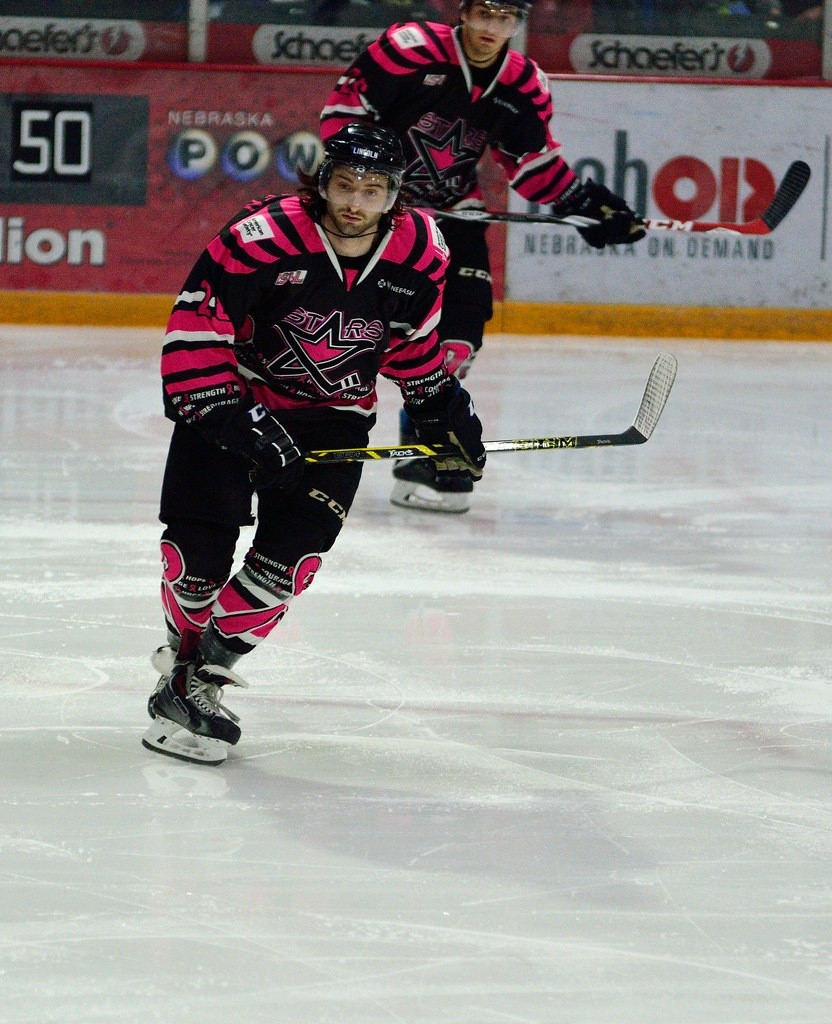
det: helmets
[318,121,402,212]
[459,0,534,38]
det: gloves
[401,374,486,482]
[553,178,647,248]
[216,387,302,475]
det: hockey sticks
[296,348,681,465]
[427,158,813,239]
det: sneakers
[141,628,250,767]
[390,408,473,515]
[147,645,178,719]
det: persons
[314,1,651,515]
[138,120,487,764]
